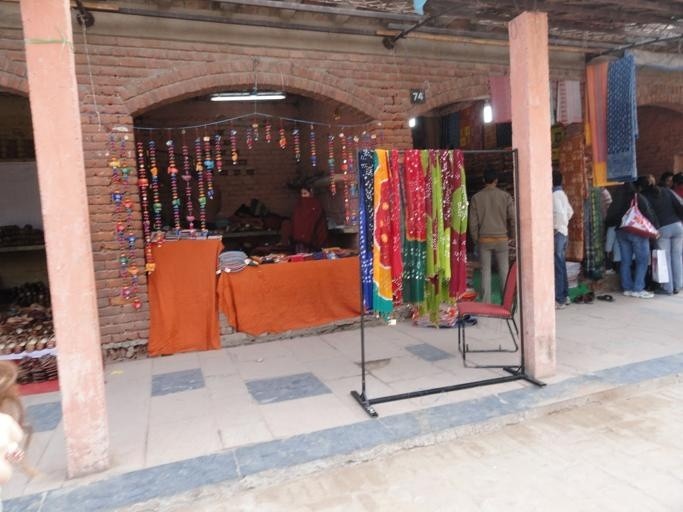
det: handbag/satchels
[651,248,670,285]
[618,193,661,239]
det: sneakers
[0,280,61,355]
[555,288,594,309]
[623,288,677,299]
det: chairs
[455,260,520,367]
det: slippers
[597,295,614,302]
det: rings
[4,448,24,463]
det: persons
[552,170,576,309]
[470,167,516,303]
[598,168,683,298]
[0,360,39,488]
[290,182,329,253]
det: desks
[146,231,361,350]
